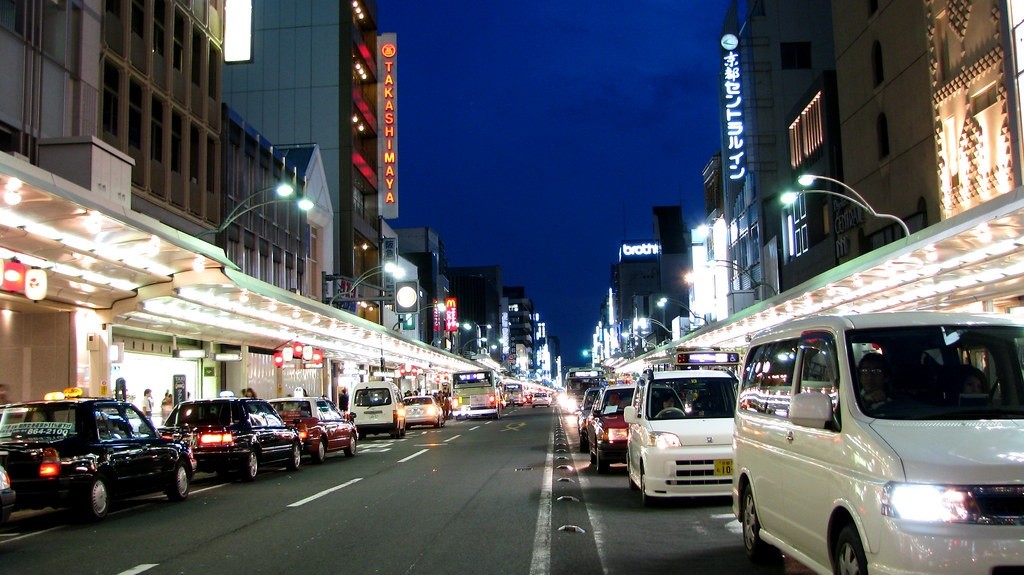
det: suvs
[0,397,195,526]
[583,382,637,474]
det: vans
[730,309,1024,575]
[622,350,742,509]
[348,381,408,441]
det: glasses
[860,369,887,376]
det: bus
[561,366,607,413]
[501,383,524,406]
[451,369,505,420]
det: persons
[856,353,890,402]
[162,392,172,426]
[246,388,256,398]
[662,393,676,414]
[142,389,154,416]
[433,387,447,402]
[339,389,349,411]
[405,390,418,397]
[951,371,984,406]
[609,392,619,406]
[509,391,515,407]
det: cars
[155,396,303,483]
[401,395,445,430]
[264,396,360,464]
[573,386,602,454]
[523,391,551,408]
